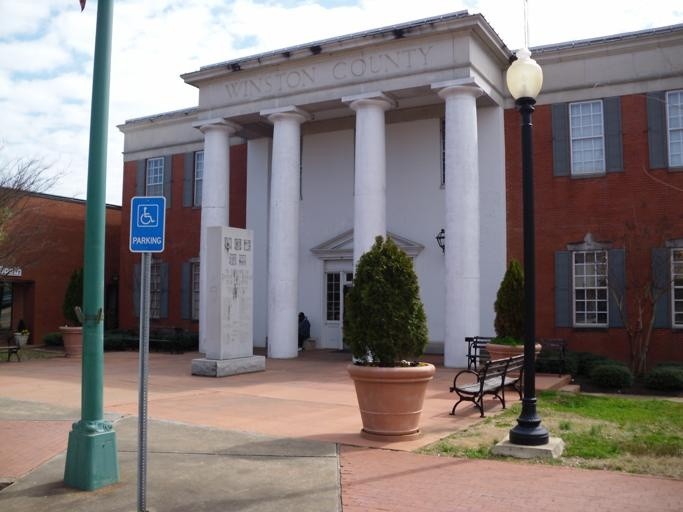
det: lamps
[435,229,445,253]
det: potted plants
[59,265,84,358]
[486,260,542,378]
[340,237,435,442]
[13,319,30,347]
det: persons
[298,311,311,353]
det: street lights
[506,48,549,445]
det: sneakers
[298,347,303,352]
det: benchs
[466,336,495,371]
[123,327,184,354]
[449,354,526,418]
[536,338,566,378]
[302,338,316,351]
[0,332,21,362]
